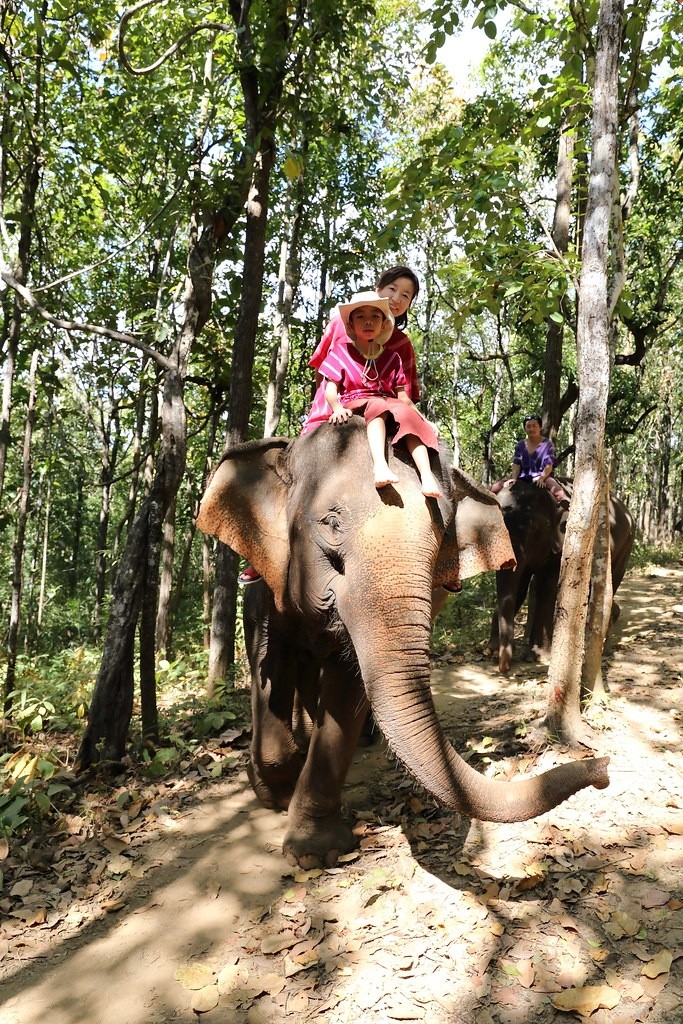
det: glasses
[525,416,539,420]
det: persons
[237,265,421,584]
[491,415,571,512]
[318,291,443,499]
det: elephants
[195,413,636,870]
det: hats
[338,291,395,345]
